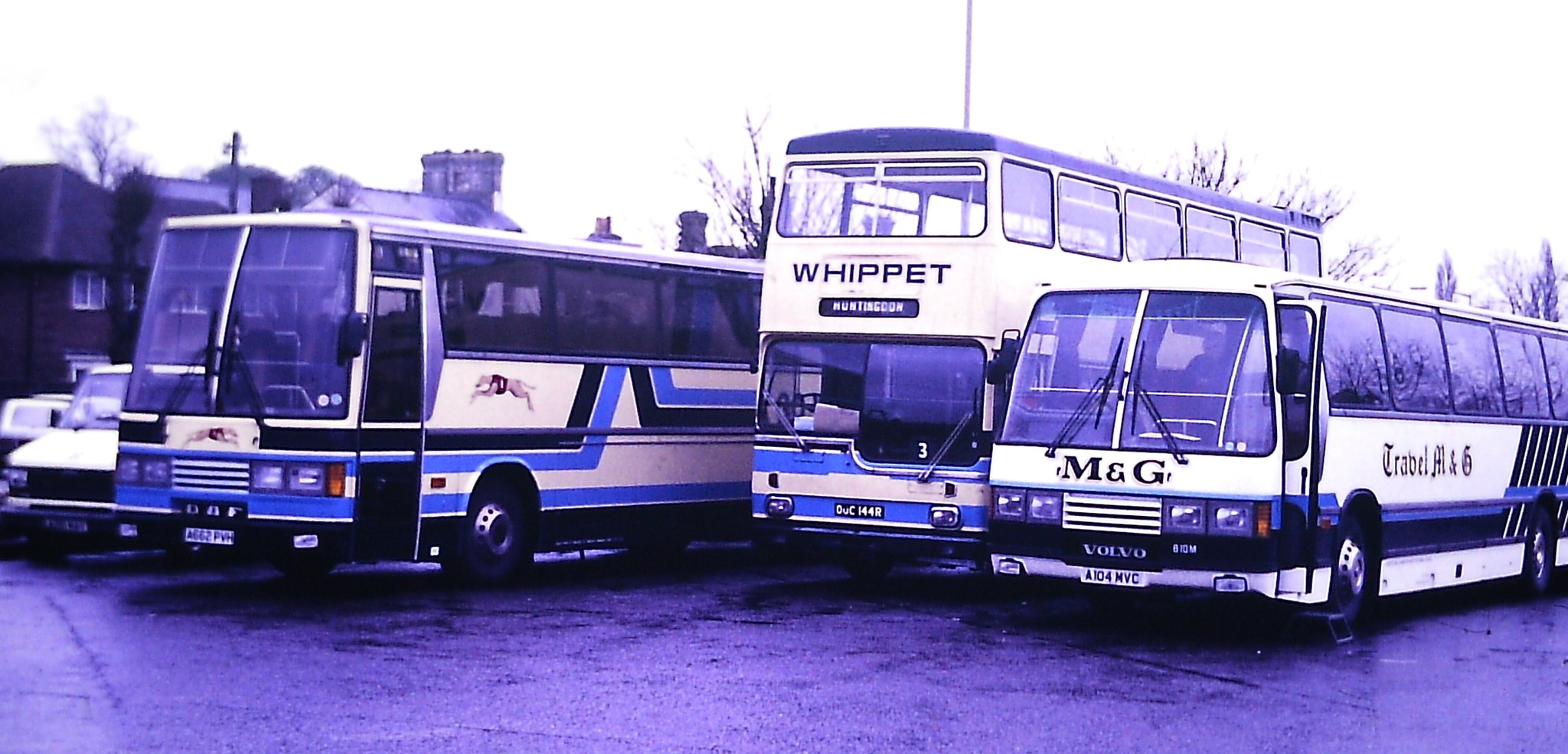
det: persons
[1186,331,1225,381]
[233,348,287,406]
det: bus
[111,215,822,595]
[983,261,1565,632]
[751,127,1327,599]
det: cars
[0,364,312,558]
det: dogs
[181,425,240,450]
[468,372,538,412]
